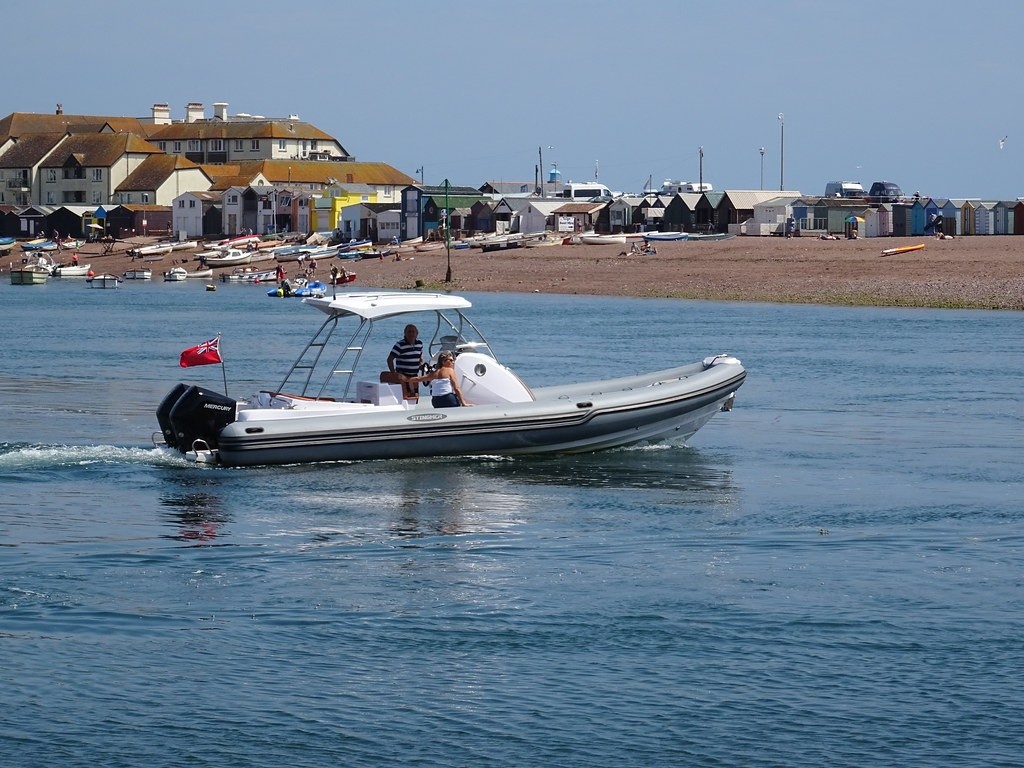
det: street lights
[759,146,765,189]
[778,112,784,190]
[539,145,555,197]
[698,145,704,193]
[439,179,453,281]
[143,193,149,236]
[416,169,423,186]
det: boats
[416,230,738,251]
[20,237,52,251]
[0,237,17,251]
[125,267,152,279]
[219,268,276,282]
[86,272,124,288]
[165,266,213,280]
[329,263,356,283]
[151,288,747,466]
[43,237,86,250]
[193,233,422,267]
[25,252,90,276]
[268,277,328,296]
[10,266,48,284]
[126,240,197,261]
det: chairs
[380,372,419,400]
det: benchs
[147,230,173,237]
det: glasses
[445,359,453,362]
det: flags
[180,336,223,368]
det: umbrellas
[86,223,103,229]
[843,216,865,223]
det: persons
[247,240,258,252]
[329,263,338,287]
[392,251,401,262]
[786,214,796,240]
[297,254,306,269]
[72,252,78,266]
[387,324,423,397]
[388,236,401,245]
[336,228,343,240]
[238,227,253,236]
[815,234,840,241]
[934,232,955,241]
[267,223,272,235]
[308,258,317,273]
[379,251,383,260]
[36,228,79,254]
[705,219,715,235]
[167,221,171,236]
[340,266,347,279]
[130,248,136,262]
[275,264,287,282]
[616,240,657,257]
[398,351,473,408]
